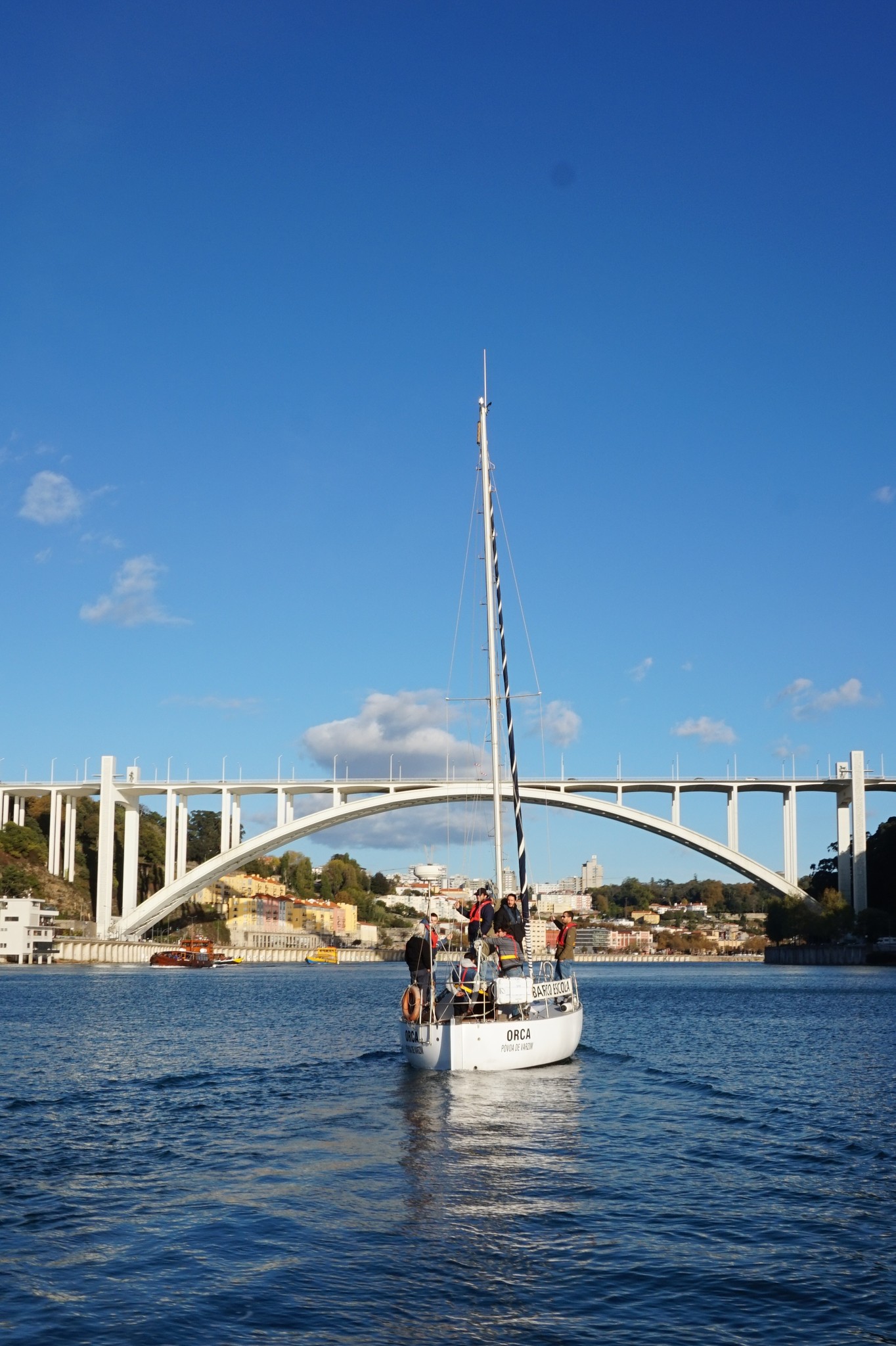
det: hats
[474,888,487,894]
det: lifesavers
[402,987,423,1021]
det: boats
[306,947,342,964]
[150,940,245,968]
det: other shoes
[523,1012,529,1018]
[557,995,572,1006]
[510,1013,522,1021]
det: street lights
[187,916,198,939]
[73,764,78,783]
[291,761,295,780]
[21,765,27,784]
[167,914,174,944]
[446,753,455,781]
[334,754,339,783]
[134,757,139,766]
[397,761,402,781]
[152,763,157,781]
[84,757,90,781]
[555,745,886,781]
[278,755,283,783]
[390,753,395,782]
[222,756,228,783]
[237,762,242,779]
[214,917,224,945]
[167,756,173,782]
[51,757,57,785]
[185,762,189,782]
[345,761,349,781]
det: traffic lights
[176,927,179,931]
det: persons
[551,911,577,1006]
[404,888,529,1021]
[161,953,180,960]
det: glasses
[561,916,570,918]
[476,894,484,897]
[498,930,505,934]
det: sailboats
[400,348,588,1080]
